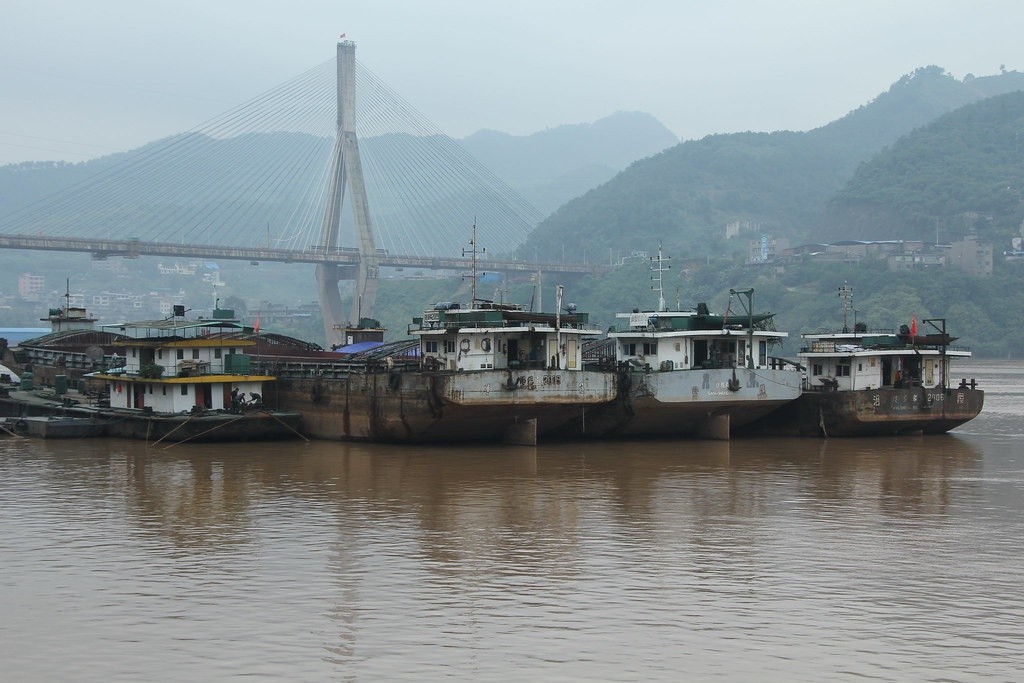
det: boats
[10,413,107,439]
[770,280,986,435]
[107,412,303,441]
[564,240,804,438]
[265,214,620,445]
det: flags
[253,318,259,334]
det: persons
[898,370,903,380]
[247,392,262,403]
[230,387,245,413]
